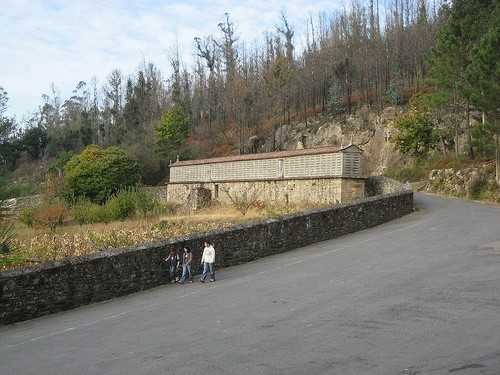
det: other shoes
[199,279,205,283]
[178,281,183,285]
[172,280,175,283]
[190,280,193,283]
[211,279,215,282]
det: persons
[199,240,216,283]
[178,247,194,283]
[165,247,180,283]
